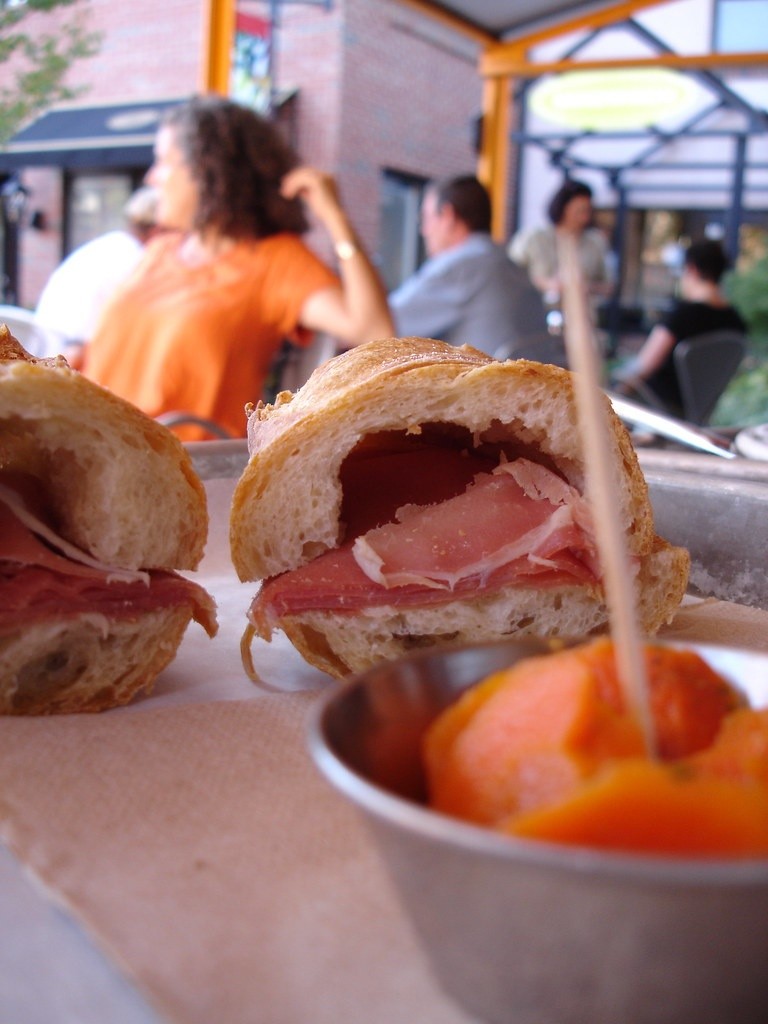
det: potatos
[414,634,768,867]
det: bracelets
[337,243,362,260]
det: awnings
[0,100,194,171]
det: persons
[387,174,547,365]
[612,240,751,450]
[33,189,171,348]
[507,179,606,312]
[78,95,396,443]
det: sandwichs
[0,322,223,715]
[228,337,691,685]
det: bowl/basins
[302,632,768,1024]
